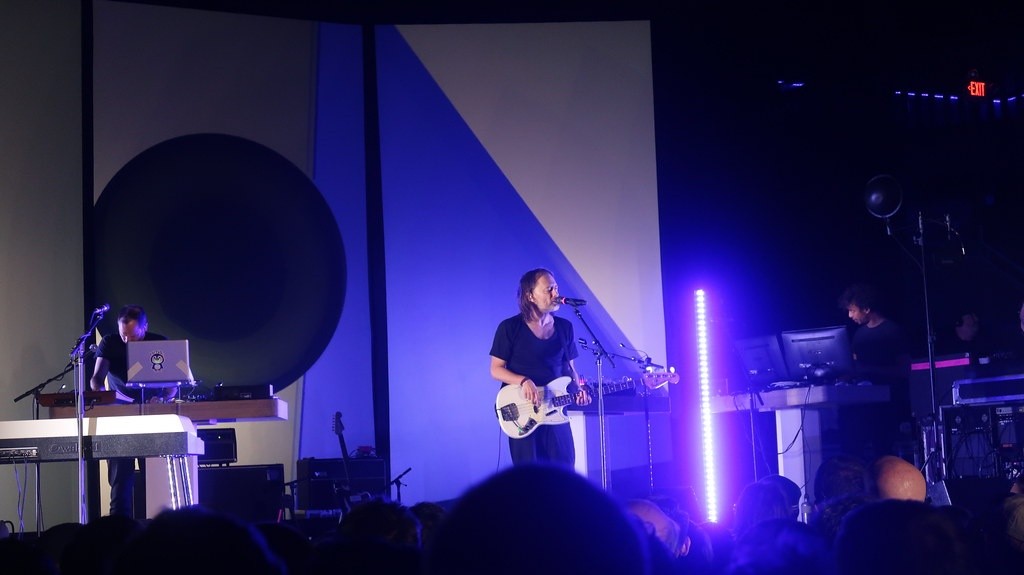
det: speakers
[296,457,391,514]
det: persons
[90,306,178,517]
[837,285,912,453]
[490,268,592,471]
[0,456,1024,575]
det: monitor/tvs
[733,335,790,386]
[781,326,850,386]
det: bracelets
[520,377,531,386]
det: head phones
[955,313,966,327]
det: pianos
[0,413,211,462]
[34,377,286,406]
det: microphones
[556,296,587,306]
[94,303,110,314]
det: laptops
[127,339,189,382]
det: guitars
[491,365,682,443]
